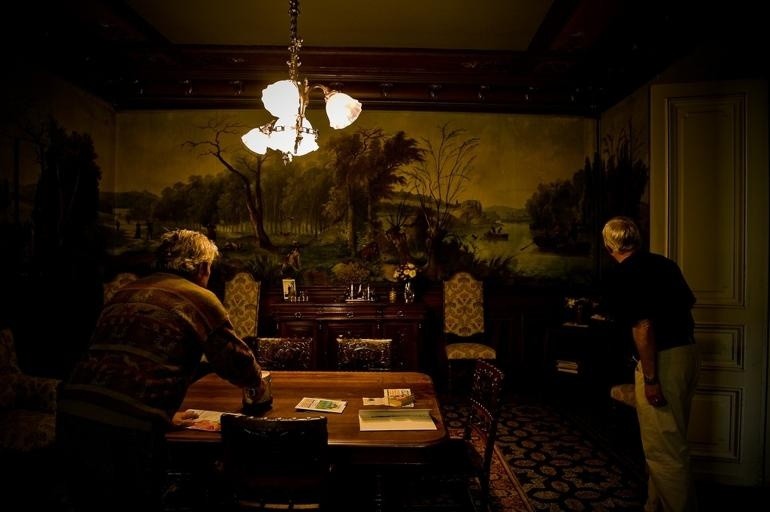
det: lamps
[240,1,363,168]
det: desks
[165,370,447,512]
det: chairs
[257,336,315,371]
[336,335,394,372]
[224,271,261,340]
[610,383,635,488]
[220,412,332,511]
[419,357,507,512]
[103,272,137,305]
[442,270,498,362]
[0,330,62,453]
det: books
[358,406,438,430]
[182,405,232,435]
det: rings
[655,400,658,402]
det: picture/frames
[282,278,296,300]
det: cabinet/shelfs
[264,292,423,372]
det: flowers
[393,262,421,283]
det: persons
[603,216,705,509]
[55,225,272,510]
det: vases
[404,283,416,304]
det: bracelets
[644,374,658,386]
